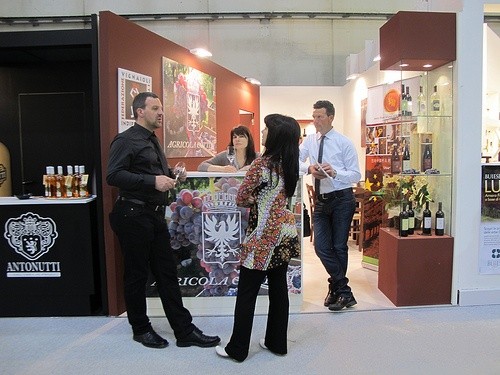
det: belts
[117,196,165,213]
[319,188,353,199]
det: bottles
[431,85,440,115]
[78,166,89,198]
[55,166,65,198]
[65,166,73,198]
[391,146,400,175]
[408,201,415,235]
[44,166,48,197]
[402,146,409,172]
[417,85,426,116]
[406,86,413,116]
[435,202,445,236]
[399,203,409,237]
[423,201,432,235]
[48,166,56,197]
[73,166,79,198]
[423,145,431,172]
[400,85,405,116]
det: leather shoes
[329,294,357,311]
[133,328,168,348]
[325,289,339,306]
[176,329,220,347]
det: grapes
[167,177,250,296]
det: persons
[298,101,361,311]
[214,113,302,363]
[197,124,263,172]
[104,92,220,348]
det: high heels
[215,345,237,361]
[259,338,284,356]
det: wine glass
[226,146,237,165]
[171,162,186,189]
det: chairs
[305,184,316,246]
[350,188,365,251]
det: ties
[315,135,326,197]
[151,135,177,203]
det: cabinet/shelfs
[378,11,457,308]
